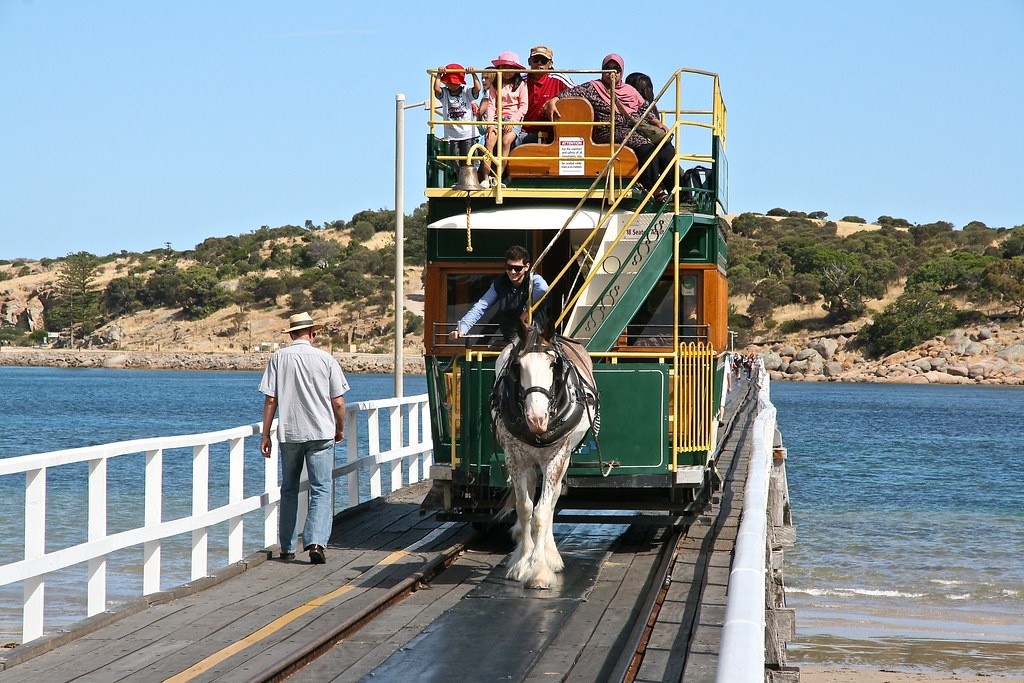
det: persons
[434,63,482,187]
[477,46,690,203]
[258,312,350,564]
[448,245,549,345]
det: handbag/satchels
[681,165,715,202]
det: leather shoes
[309,544,325,564]
[280,552,294,559]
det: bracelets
[260,434,270,439]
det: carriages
[421,64,734,590]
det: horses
[489,335,600,590]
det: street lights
[393,95,444,482]
[728,331,738,354]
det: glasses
[505,264,527,272]
[531,57,548,64]
[313,332,316,338]
[604,66,620,74]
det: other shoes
[481,181,489,188]
[656,192,673,204]
[501,183,506,188]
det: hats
[281,312,323,333]
[492,52,526,69]
[531,46,553,60]
[441,64,466,85]
